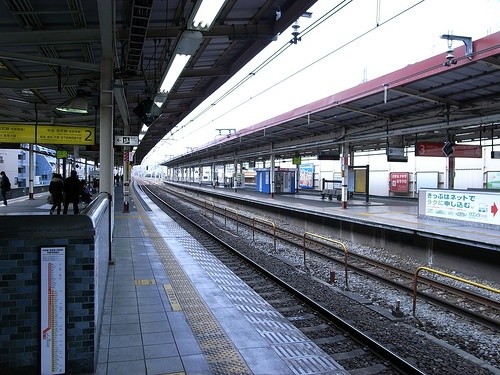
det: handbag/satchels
[47,195,53,204]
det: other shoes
[50,210,53,215]
[1,204,6,206]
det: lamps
[157,30,204,93]
[55,97,88,113]
[131,95,168,155]
[185,0,229,32]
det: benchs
[319,188,342,201]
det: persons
[49,170,96,215]
[0,171,10,206]
[114,173,123,186]
